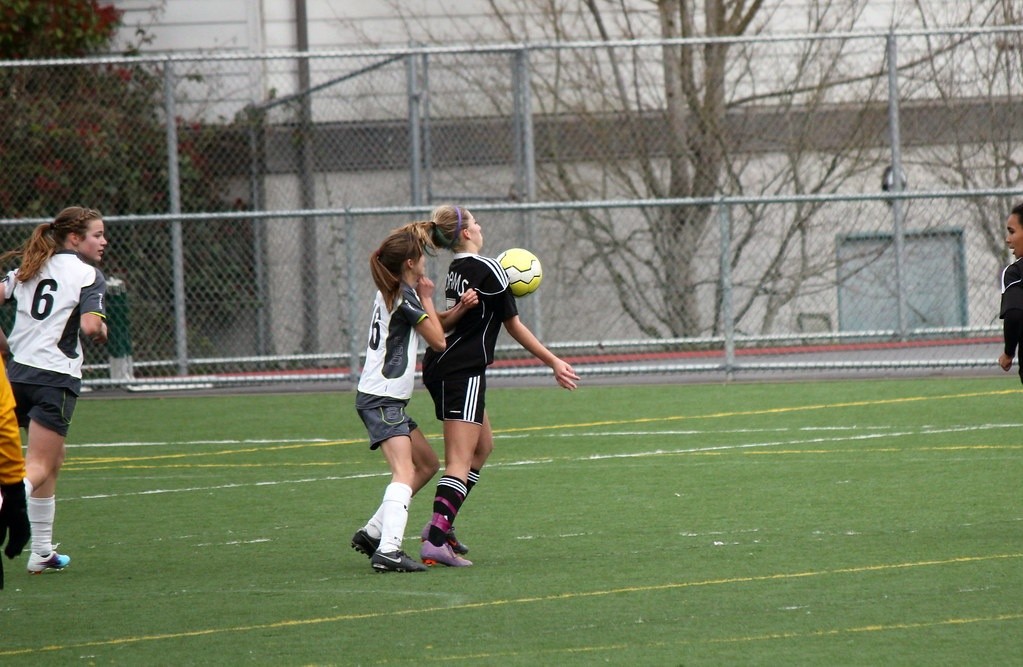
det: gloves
[0,480,31,560]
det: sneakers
[421,520,469,555]
[350,526,381,559]
[371,548,429,573]
[26,543,72,575]
[420,540,473,567]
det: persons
[998,203,1023,385]
[0,207,108,573]
[402,205,580,567]
[350,234,479,574]
[0,352,32,590]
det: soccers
[496,246,544,297]
